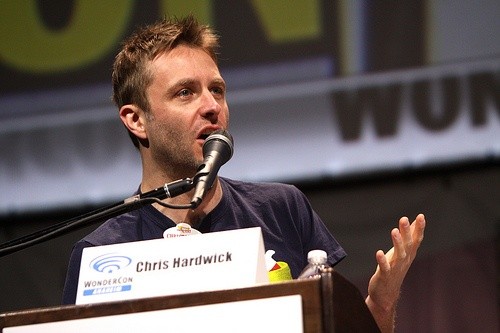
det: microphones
[188,128,235,210]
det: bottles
[298,250,331,279]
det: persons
[58,12,428,333]
